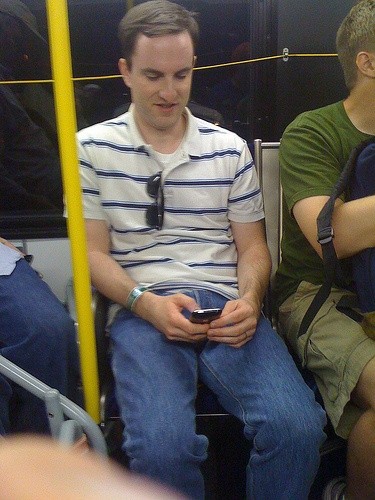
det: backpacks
[296,138,375,340]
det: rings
[245,331,248,339]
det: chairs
[69,136,286,420]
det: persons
[0,434,218,500]
[62,2,328,500]
[0,238,78,436]
[0,0,252,238]
[273,0,375,500]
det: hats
[0,0,48,45]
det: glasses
[146,171,164,230]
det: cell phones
[189,308,222,324]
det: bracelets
[128,287,151,314]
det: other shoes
[323,476,347,500]
